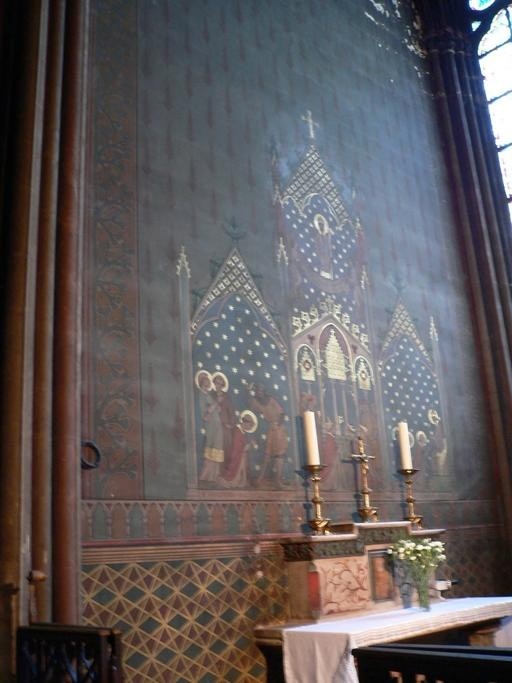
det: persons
[312,212,334,278]
[195,369,289,488]
[390,369,448,493]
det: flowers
[387,536,449,577]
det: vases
[416,580,431,610]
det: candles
[396,420,412,468]
[302,409,320,463]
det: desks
[252,597,511,683]
